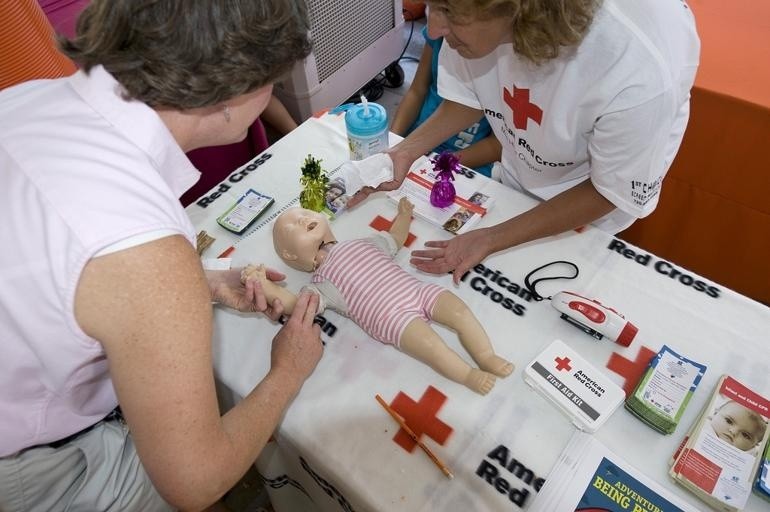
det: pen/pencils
[375,395,454,480]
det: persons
[258,94,298,134]
[0,0,325,512]
[710,400,767,451]
[390,25,503,179]
[345,0,700,286]
[240,196,515,397]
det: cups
[346,103,388,158]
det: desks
[179,103,769,511]
